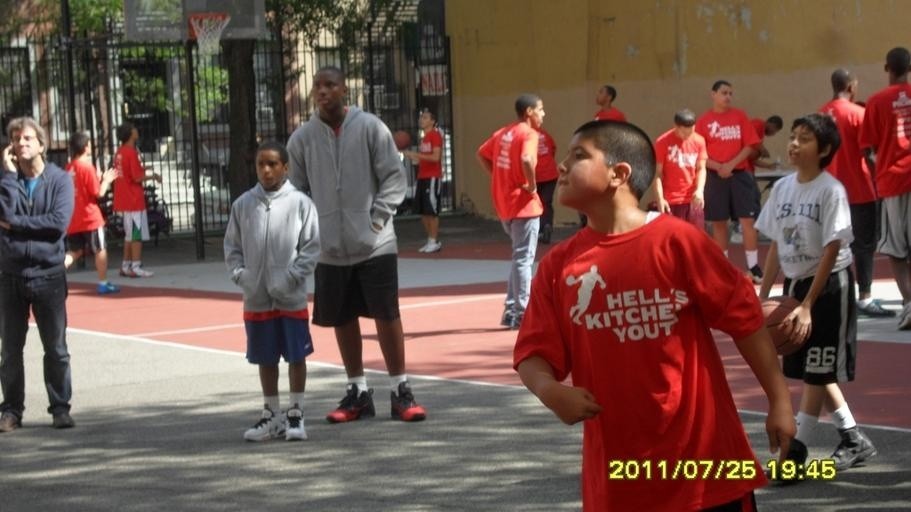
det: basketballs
[761,296,812,355]
[393,130,411,149]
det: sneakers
[818,426,877,472]
[96,265,154,294]
[501,308,524,329]
[766,439,807,482]
[391,380,426,421]
[749,264,911,332]
[419,242,441,252]
[326,383,376,423]
[244,409,286,441]
[286,408,307,441]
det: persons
[223,143,321,441]
[513,118,797,512]
[0,116,75,430]
[403,107,443,254]
[756,115,880,488]
[477,47,911,329]
[63,134,121,294]
[283,66,427,424]
[112,122,154,278]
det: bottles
[776,155,781,174]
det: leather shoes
[52,413,74,428]
[0,412,23,432]
